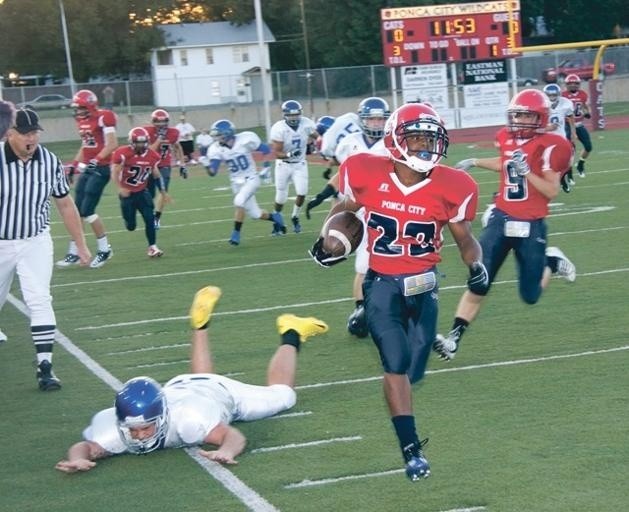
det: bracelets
[264,162,270,167]
[95,156,102,161]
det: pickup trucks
[543,59,615,86]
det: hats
[11,108,45,133]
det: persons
[315,112,362,180]
[112,127,171,258]
[270,101,319,235]
[192,120,288,245]
[306,97,389,309]
[143,109,189,230]
[1,109,92,388]
[314,105,490,480]
[175,116,212,166]
[543,83,579,193]
[57,91,117,268]
[434,88,577,363]
[563,74,593,185]
[55,288,329,475]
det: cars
[13,94,78,111]
[506,74,538,87]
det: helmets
[71,89,98,121]
[356,95,392,138]
[383,103,449,173]
[115,376,170,454]
[543,83,561,106]
[506,89,551,138]
[316,115,338,138]
[564,74,581,93]
[211,120,236,146]
[280,99,306,128]
[128,109,170,155]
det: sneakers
[560,164,584,193]
[55,243,114,269]
[401,438,431,483]
[37,359,62,391]
[188,286,222,328]
[148,245,164,257]
[277,314,328,343]
[229,212,301,246]
[348,306,368,338]
[433,333,457,363]
[544,247,576,282]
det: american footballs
[322,210,363,256]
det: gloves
[467,261,490,296]
[179,164,189,179]
[84,161,98,175]
[198,156,210,167]
[305,194,323,219]
[452,158,474,171]
[308,237,348,269]
[508,148,530,175]
[323,168,330,180]
